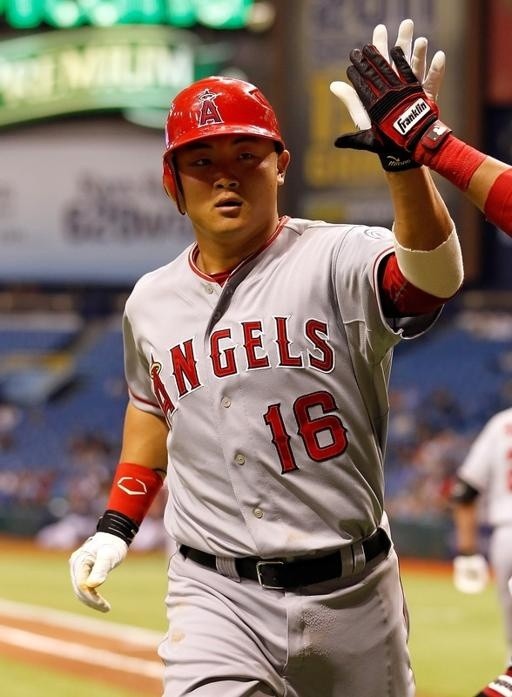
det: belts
[178,526,391,590]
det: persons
[437,403,512,669]
[68,20,447,696]
[334,38,512,242]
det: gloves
[451,552,491,597]
[328,17,453,173]
[67,508,139,613]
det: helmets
[160,75,287,215]
